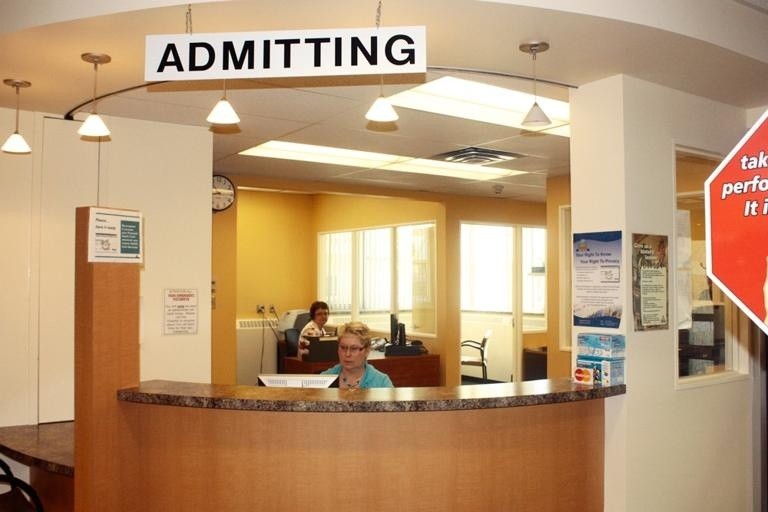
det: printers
[301,335,340,362]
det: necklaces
[341,372,362,388]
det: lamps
[519,41,553,126]
[0,79,32,153]
[78,52,111,136]
[206,79,241,124]
[364,74,399,122]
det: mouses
[412,340,422,345]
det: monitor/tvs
[257,373,340,389]
[390,315,408,345]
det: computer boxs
[384,342,421,357]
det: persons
[294,300,330,361]
[319,321,397,389]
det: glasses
[338,342,366,351]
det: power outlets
[259,305,265,313]
[270,305,274,313]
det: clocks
[212,174,236,211]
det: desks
[283,350,440,387]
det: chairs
[461,335,489,384]
[285,329,299,356]
[0,459,44,512]
